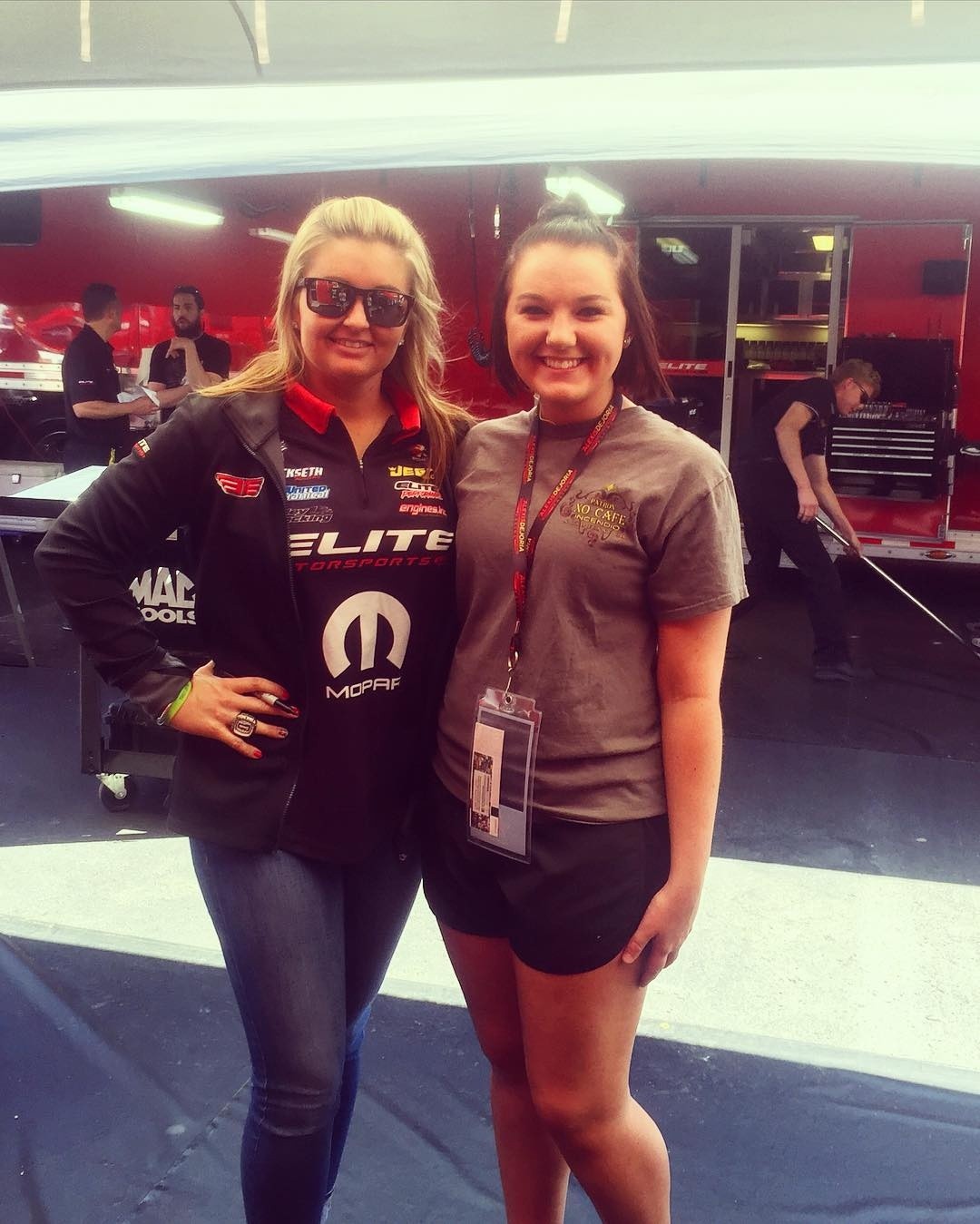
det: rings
[231,712,256,737]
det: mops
[812,515,980,662]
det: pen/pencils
[221,670,297,717]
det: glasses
[176,285,200,294]
[855,381,869,403]
[297,277,414,327]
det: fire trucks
[2,160,980,570]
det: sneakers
[815,661,874,685]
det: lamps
[545,166,625,216]
[248,228,295,244]
[107,186,225,226]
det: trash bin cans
[80,567,196,812]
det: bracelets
[158,681,192,728]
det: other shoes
[61,615,72,629]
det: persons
[33,194,484,1224]
[733,358,880,682]
[147,285,231,423]
[61,283,159,474]
[418,199,751,1224]
